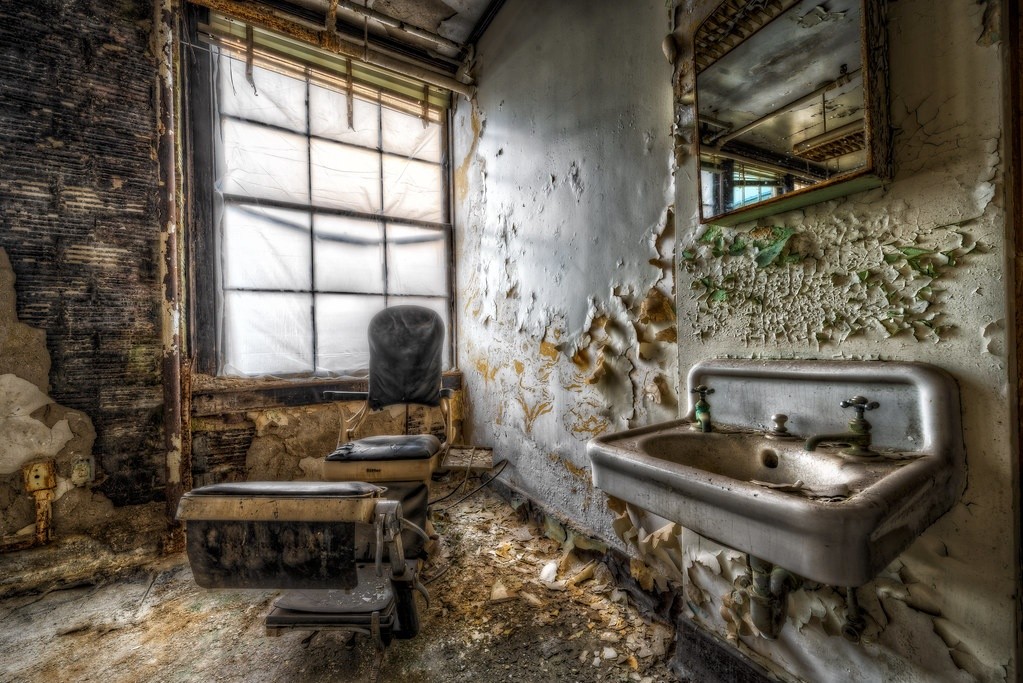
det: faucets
[689,383,717,434]
[804,395,883,461]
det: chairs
[319,305,454,633]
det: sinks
[587,417,961,589]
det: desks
[176,482,416,637]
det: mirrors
[693,0,891,227]
